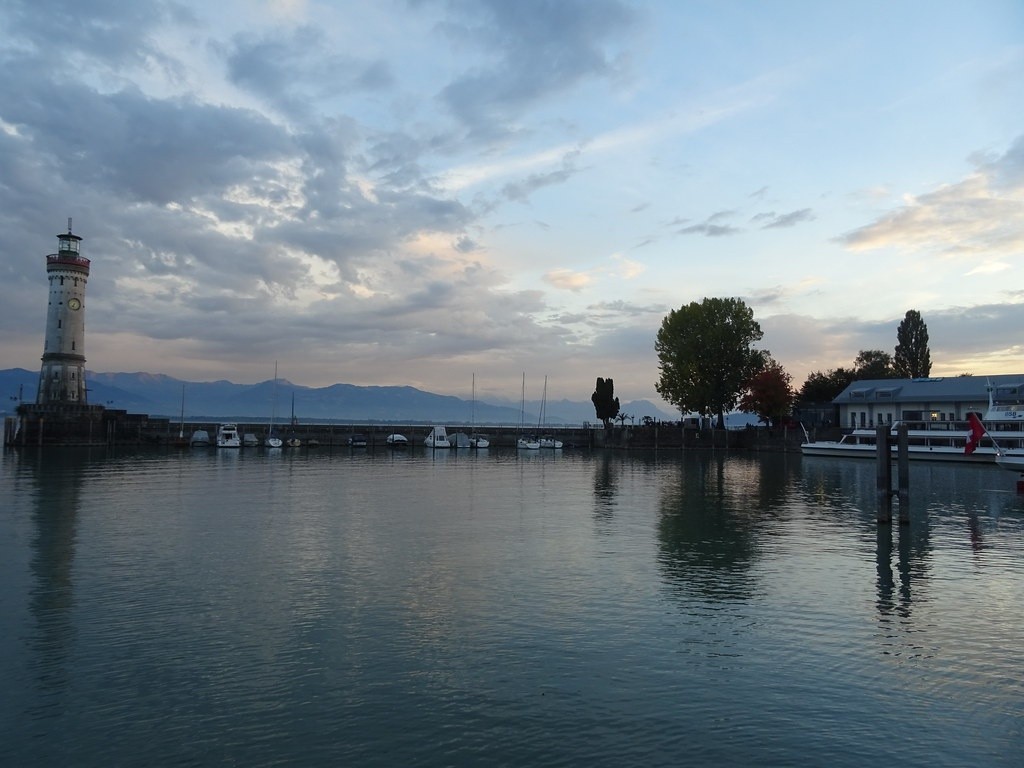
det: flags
[965,415,986,456]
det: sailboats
[469,372,490,448]
[517,371,540,449]
[286,392,302,448]
[264,360,282,447]
[536,374,564,448]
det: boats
[244,434,259,448]
[308,438,320,447]
[217,424,242,448]
[190,430,211,448]
[386,434,407,447]
[969,405,1023,471]
[425,426,451,448]
[349,434,368,447]
[448,433,471,448]
[798,378,1024,463]
